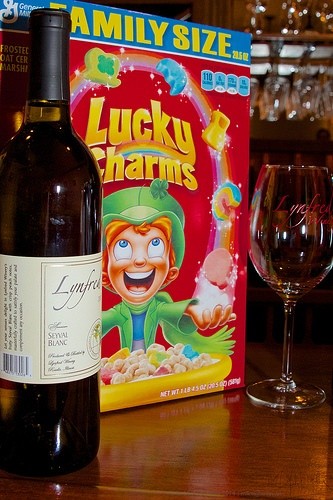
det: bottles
[0,8,104,475]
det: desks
[0,342,333,500]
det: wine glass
[246,164,333,409]
[244,0,333,122]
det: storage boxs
[0,0,251,421]
